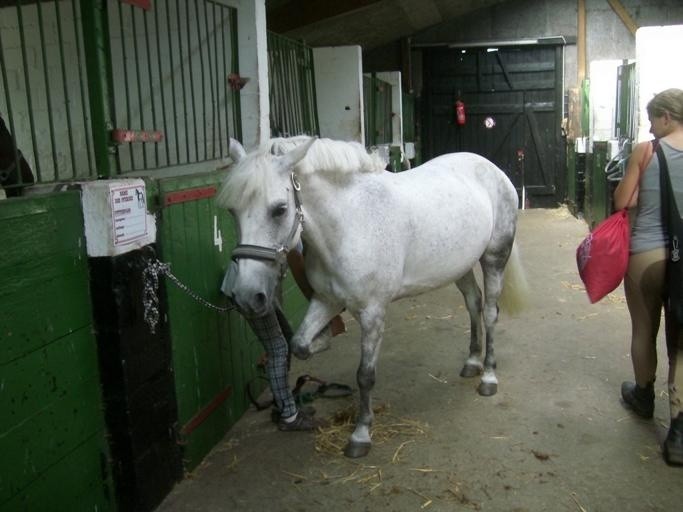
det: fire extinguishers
[455,90,465,126]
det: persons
[613,88,683,466]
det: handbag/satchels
[575,210,630,305]
[662,218,682,316]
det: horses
[211,133,528,459]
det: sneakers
[269,407,329,429]
[620,380,657,419]
[661,416,682,466]
